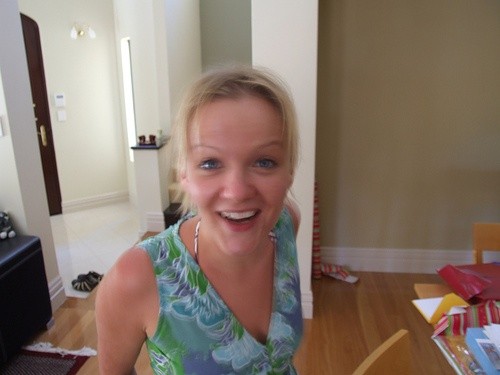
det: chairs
[474,221,500,266]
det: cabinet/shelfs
[0,233,54,352]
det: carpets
[2,349,89,375]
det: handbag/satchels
[437,262,500,304]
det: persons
[96,64,305,375]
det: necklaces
[194,218,277,261]
